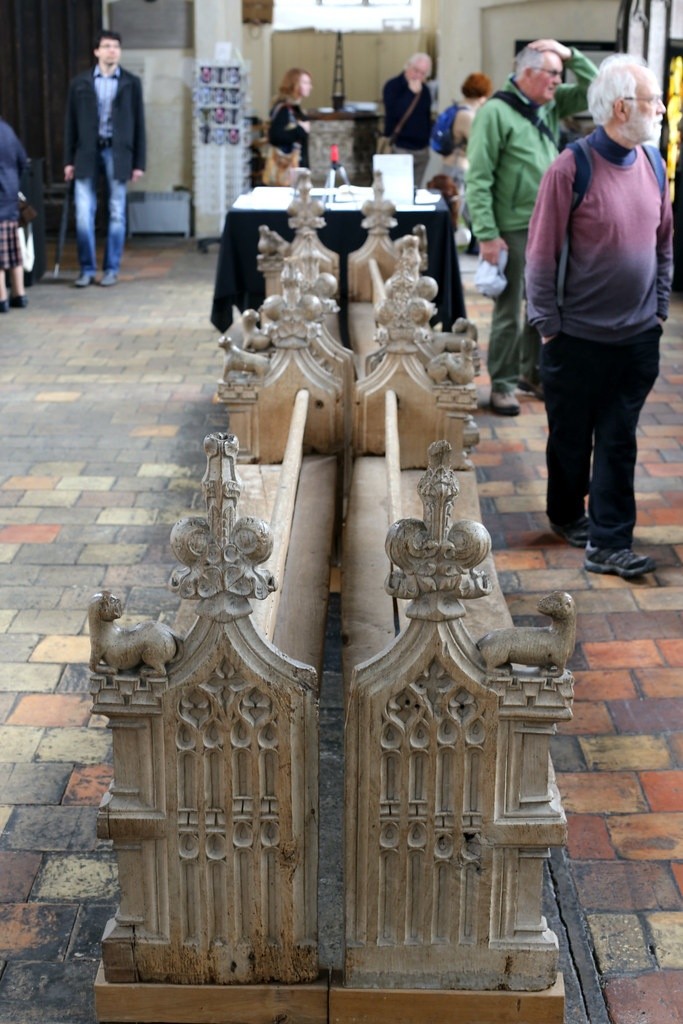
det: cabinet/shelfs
[193,60,250,250]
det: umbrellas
[53,178,71,277]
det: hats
[473,248,508,297]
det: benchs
[88,171,574,1024]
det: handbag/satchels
[17,195,38,226]
[375,136,392,155]
[261,102,301,187]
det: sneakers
[584,540,655,578]
[549,515,591,546]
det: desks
[305,110,386,183]
[211,184,466,334]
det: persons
[0,120,31,313]
[465,39,601,415]
[267,68,313,168]
[63,29,148,287]
[382,53,491,256]
[525,55,673,579]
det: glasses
[612,92,665,107]
[532,64,564,80]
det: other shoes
[100,272,118,286]
[74,273,95,286]
[8,294,28,308]
[0,300,9,313]
[517,373,547,402]
[489,390,520,416]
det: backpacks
[429,103,469,157]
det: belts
[98,138,112,148]
